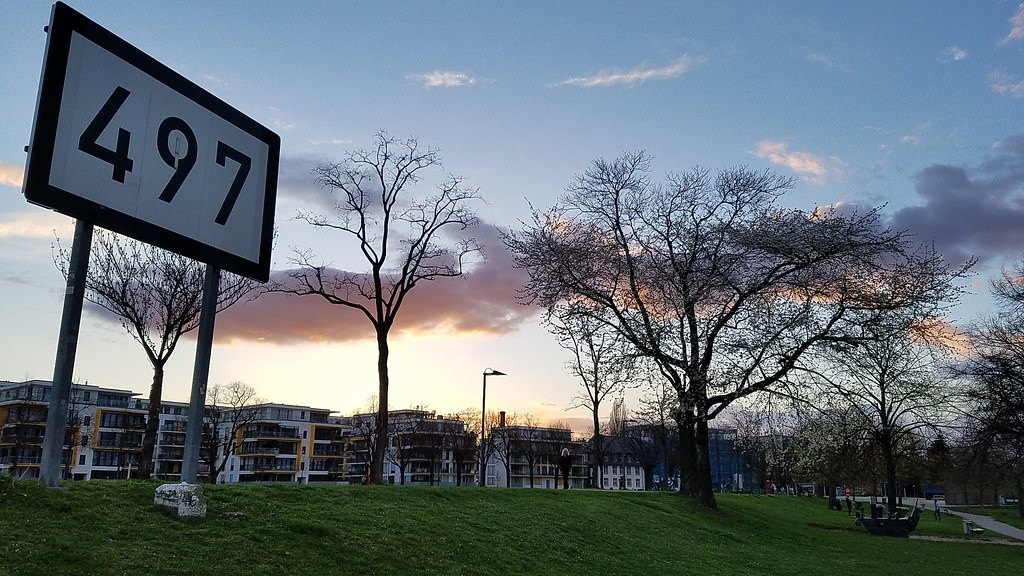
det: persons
[934,499,941,521]
[560,448,572,490]
[846,494,852,517]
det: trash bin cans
[963,520,973,534]
[896,506,910,518]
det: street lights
[478,367,507,487]
